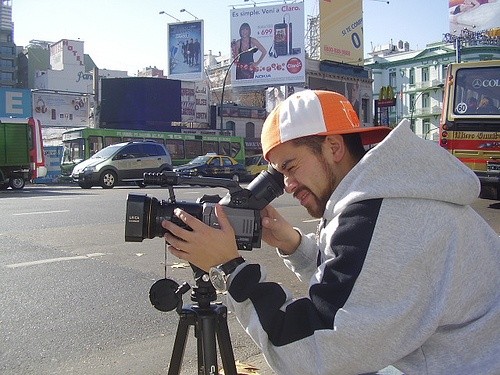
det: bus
[56,127,246,176]
[438,59,500,200]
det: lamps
[180,9,199,21]
[158,11,180,22]
[244,0,256,7]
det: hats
[261,90,393,162]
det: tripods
[166,258,241,375]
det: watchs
[209,255,245,293]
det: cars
[173,152,245,182]
[246,153,268,181]
[69,139,174,189]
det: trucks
[0,117,46,190]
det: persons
[162,90,500,375]
[465,96,493,113]
[233,23,268,80]
[181,38,200,68]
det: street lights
[220,46,258,136]
[411,83,444,129]
